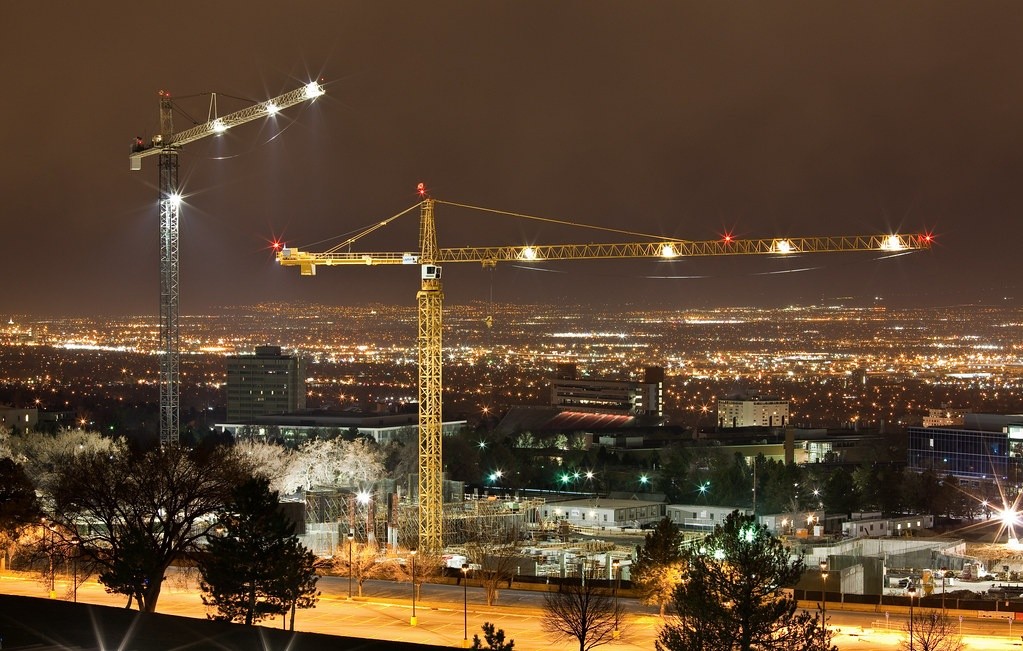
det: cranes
[129,81,326,453]
[279,195,931,582]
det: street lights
[909,585,916,651]
[347,533,353,602]
[821,561,830,645]
[49,524,58,590]
[408,546,418,625]
[461,563,470,641]
[70,537,79,602]
